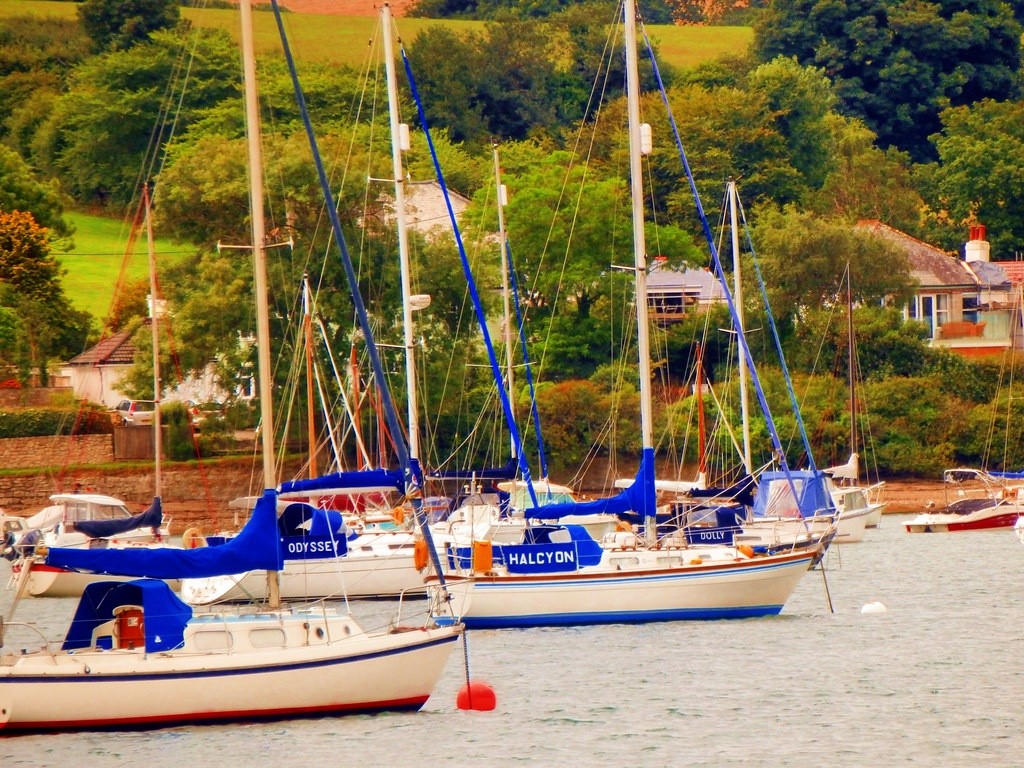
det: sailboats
[0,0,884,604]
[409,0,830,630]
[0,1,475,736]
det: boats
[902,465,1024,535]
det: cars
[192,410,227,430]
[113,400,155,426]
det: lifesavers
[182,528,203,547]
